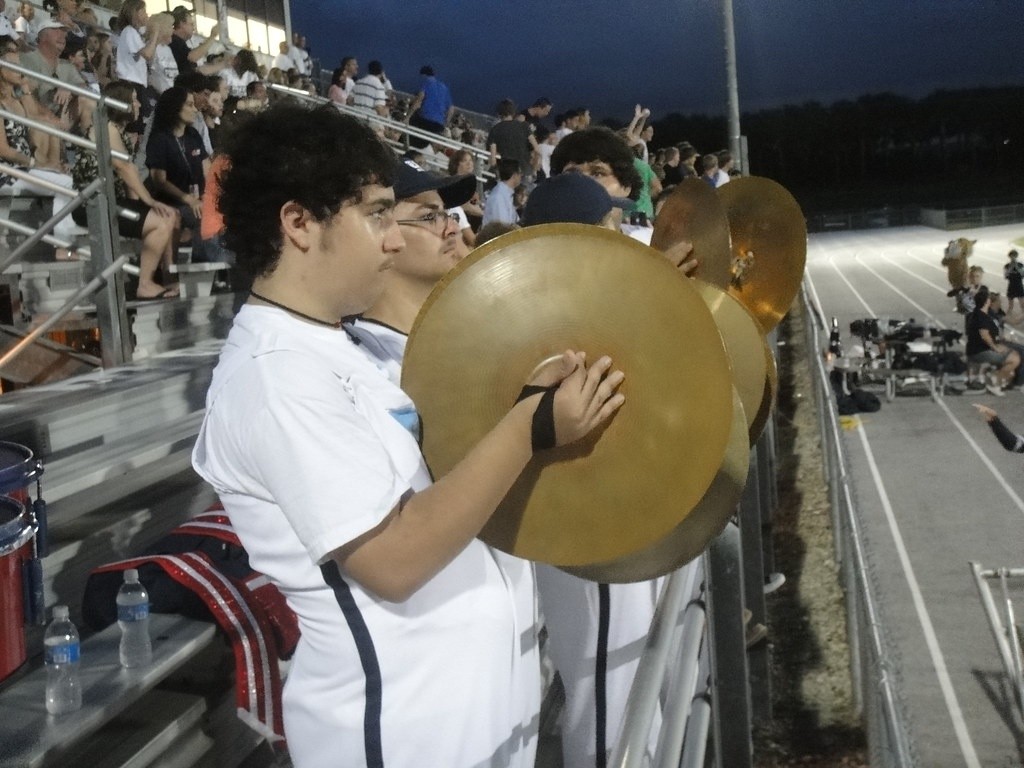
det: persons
[941,237,976,287]
[1002,250,1024,317]
[988,291,1004,334]
[965,291,1021,396]
[0,0,744,392]
[948,265,988,313]
[191,102,626,768]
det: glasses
[396,212,460,231]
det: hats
[392,156,478,210]
[207,52,225,63]
[522,170,637,228]
[36,21,69,36]
[173,5,196,16]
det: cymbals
[399,173,807,584]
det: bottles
[42,606,83,717]
[116,569,154,667]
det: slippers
[136,290,179,301]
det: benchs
[2,120,496,766]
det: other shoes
[986,382,1005,397]
